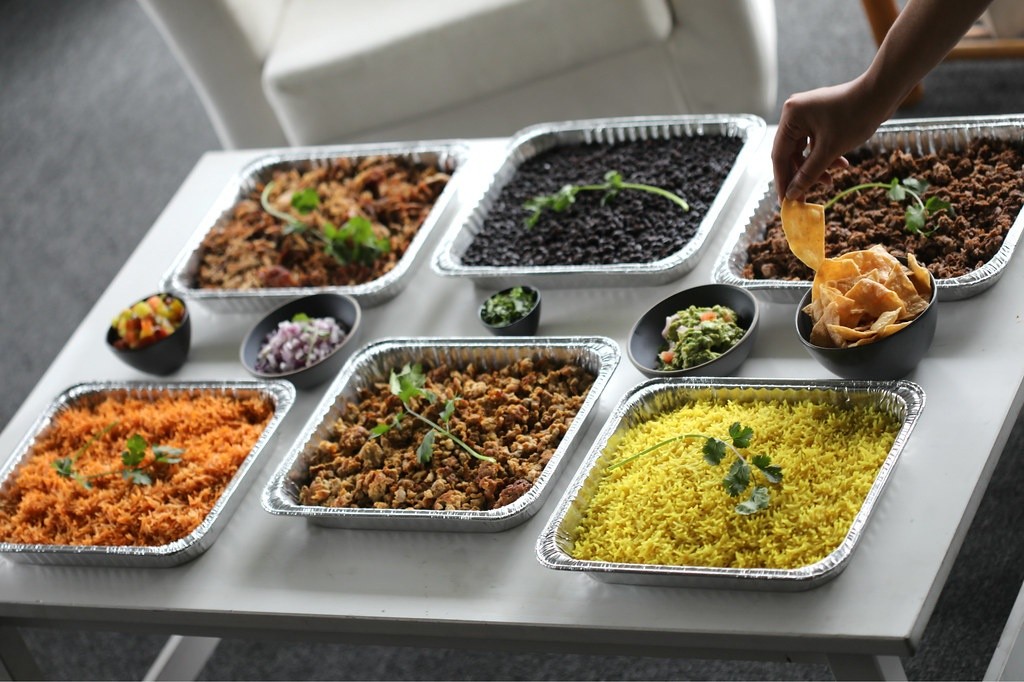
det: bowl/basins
[481,286,540,335]
[105,294,195,376]
[238,293,360,388]
[629,283,760,379]
[794,254,940,384]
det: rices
[573,399,902,570]
[0,391,273,548]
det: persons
[770,0,990,209]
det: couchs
[143,0,776,153]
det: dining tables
[0,140,1022,680]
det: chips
[780,196,931,349]
